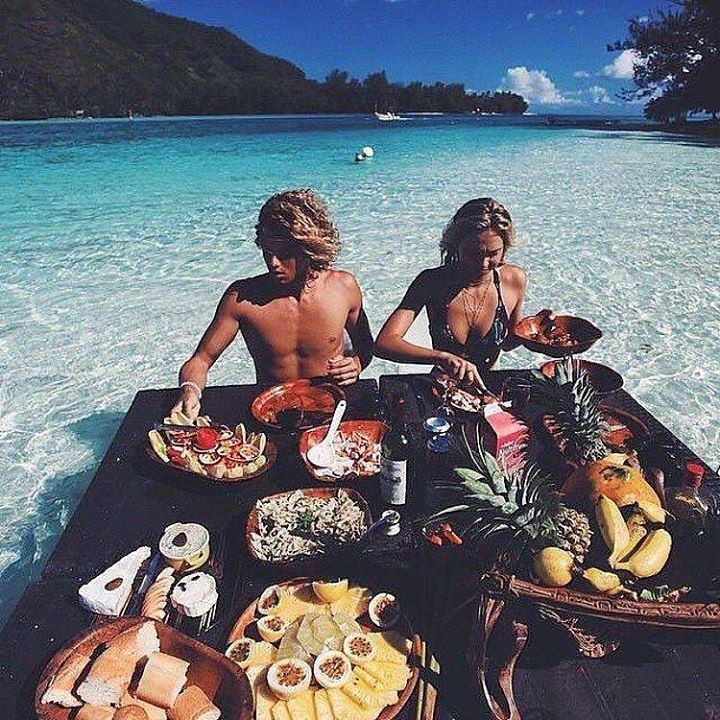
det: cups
[502,377,531,413]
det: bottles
[378,393,415,513]
[671,464,709,528]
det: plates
[246,485,373,569]
[297,419,392,484]
[145,426,278,484]
[226,576,422,720]
[34,616,255,720]
[250,377,346,430]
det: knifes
[129,553,158,616]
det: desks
[0,367,720,720]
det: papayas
[556,460,664,509]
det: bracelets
[180,380,202,399]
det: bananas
[594,494,676,577]
[604,448,641,466]
[570,566,621,592]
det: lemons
[312,578,348,603]
[531,546,578,587]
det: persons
[169,186,372,430]
[373,197,557,392]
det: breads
[141,567,176,620]
[42,622,222,719]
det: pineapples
[427,424,607,568]
[247,586,413,720]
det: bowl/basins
[533,405,650,455]
[513,316,603,358]
[159,523,210,571]
[539,359,625,399]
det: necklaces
[460,280,492,328]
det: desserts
[77,523,220,616]
